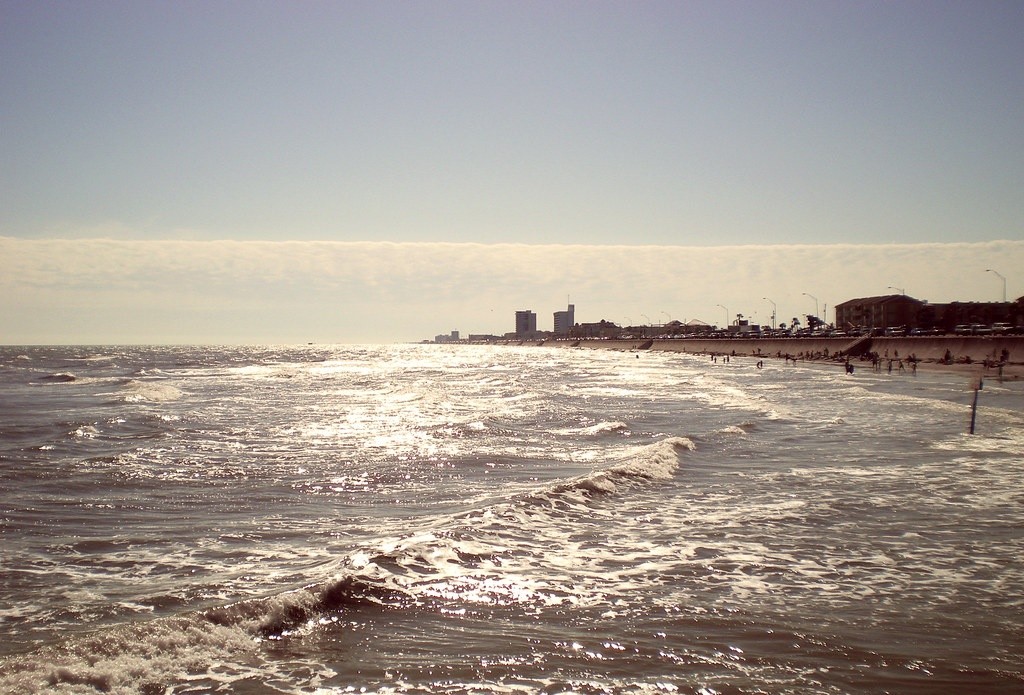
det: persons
[548,327,1024,377]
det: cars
[641,330,823,339]
[830,322,1015,337]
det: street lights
[640,314,651,326]
[660,310,671,324]
[717,304,729,327]
[623,315,634,327]
[763,297,777,328]
[802,292,819,317]
[985,269,1007,300]
